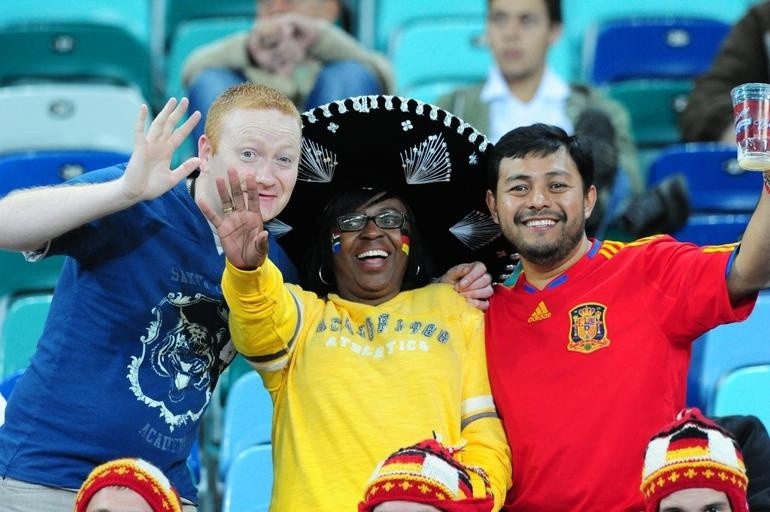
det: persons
[484,123,769,512]
[0,83,496,512]
[197,94,513,512]
[178,0,396,164]
[685,1,769,144]
[430,0,643,231]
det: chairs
[342,0,491,107]
[546,1,763,245]
[165,1,256,170]
[1,0,166,424]
[685,288,768,436]
[189,351,274,512]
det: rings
[221,206,235,213]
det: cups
[730,84,770,172]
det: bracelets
[761,174,768,194]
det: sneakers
[608,174,688,238]
[577,108,618,234]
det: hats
[275,94,521,299]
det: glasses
[335,211,411,231]
